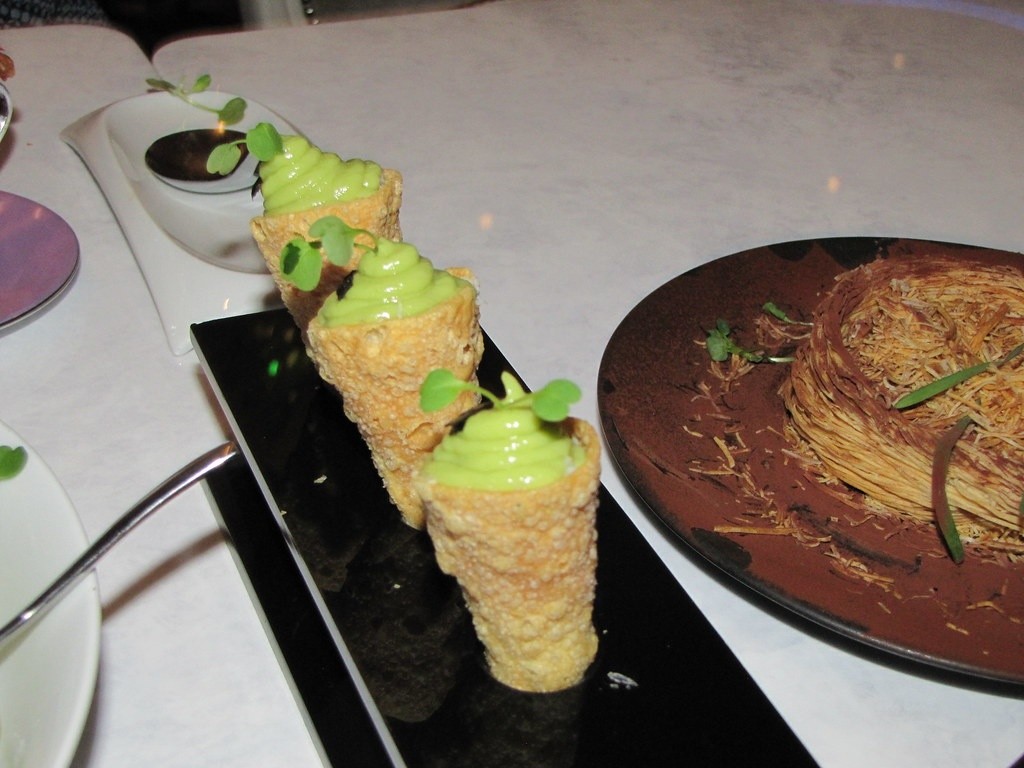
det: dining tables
[0,0,1024,768]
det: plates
[185,291,824,768]
[0,186,80,331]
[3,425,107,767]
[596,235,1023,689]
[64,94,320,359]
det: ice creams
[248,131,603,693]
